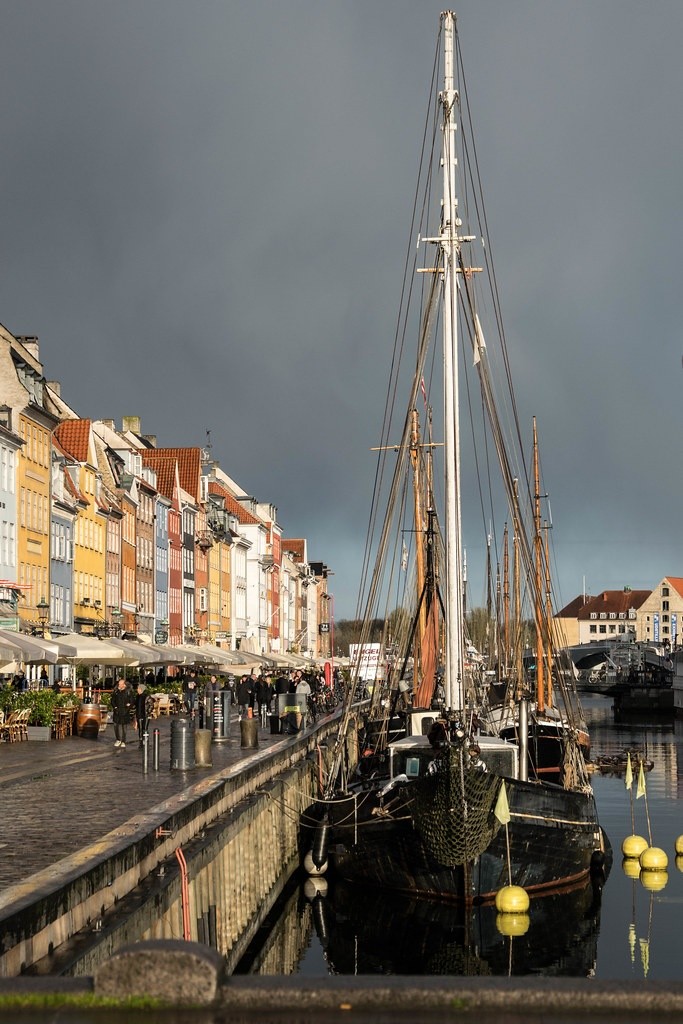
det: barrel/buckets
[99,704,109,732]
[76,703,101,738]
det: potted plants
[0,673,57,741]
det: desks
[52,710,77,735]
[53,713,68,736]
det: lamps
[81,598,90,605]
[93,600,101,607]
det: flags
[636,760,646,799]
[494,779,511,824]
[625,752,633,790]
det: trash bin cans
[269,716,283,733]
[169,717,196,771]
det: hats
[469,744,481,753]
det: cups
[248,707,252,718]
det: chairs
[0,708,32,744]
[154,698,176,716]
[52,705,79,740]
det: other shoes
[138,743,143,749]
[238,715,242,722]
[113,740,121,747]
[120,742,126,747]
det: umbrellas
[0,627,354,698]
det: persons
[111,679,154,747]
[11,675,22,688]
[51,681,61,693]
[173,667,347,723]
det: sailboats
[296,11,612,976]
